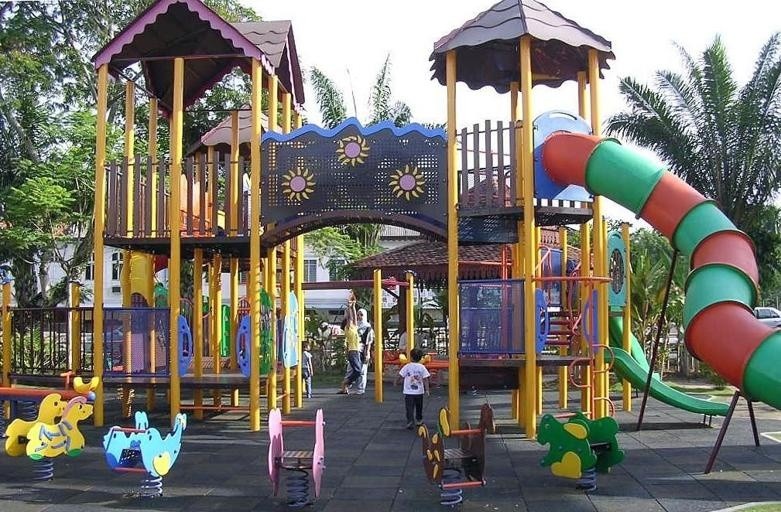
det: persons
[342,307,376,396]
[301,340,315,399]
[339,292,364,393]
[392,348,432,429]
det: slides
[604,304,661,391]
[540,130,781,409]
[602,346,730,416]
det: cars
[754,306,781,327]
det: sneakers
[415,419,425,426]
[406,420,415,430]
[355,391,365,395]
[339,377,352,394]
[307,394,313,399]
